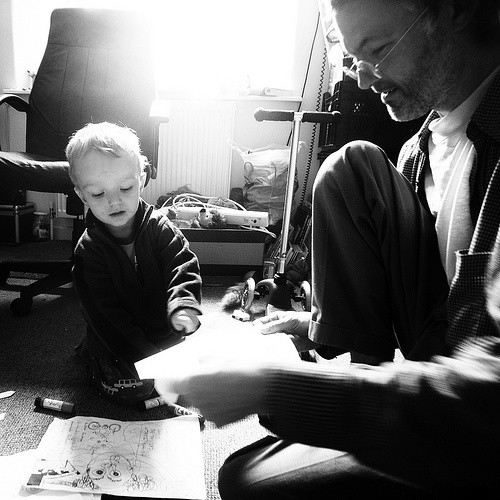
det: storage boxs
[0,202,35,243]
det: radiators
[54,100,237,218]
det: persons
[151,1,500,500]
[65,121,202,383]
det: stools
[177,225,281,269]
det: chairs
[0,9,169,318]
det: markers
[167,403,205,424]
[34,396,77,414]
[137,395,167,411]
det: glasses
[341,7,437,80]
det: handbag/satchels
[227,140,308,224]
